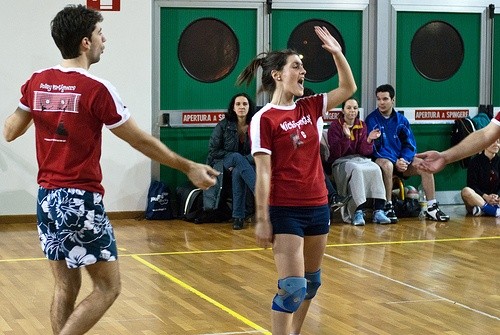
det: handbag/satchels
[145,181,173,220]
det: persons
[3,4,221,334]
[205,94,257,230]
[328,97,392,226]
[242,24,357,335]
[462,139,500,218]
[298,87,353,219]
[413,110,500,174]
[365,85,450,222]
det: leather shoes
[234,219,244,229]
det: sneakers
[426,202,450,222]
[473,206,483,216]
[352,210,365,226]
[373,210,391,224]
[385,204,398,223]
